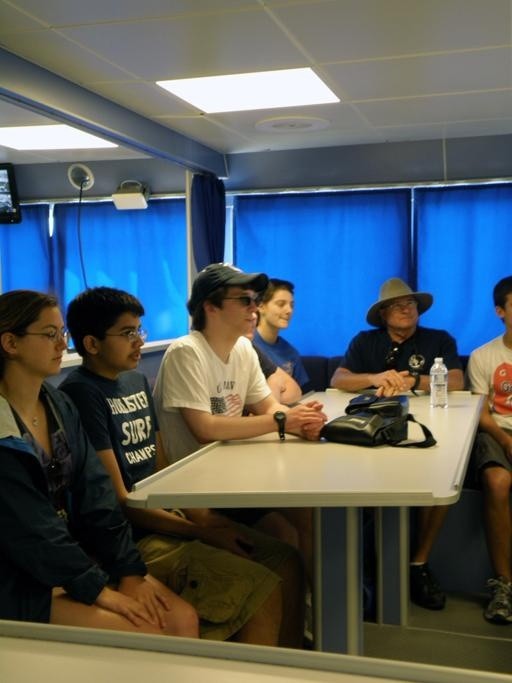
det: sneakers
[482,574,512,625]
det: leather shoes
[406,562,447,611]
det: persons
[54,285,306,646]
[151,262,313,584]
[252,275,312,389]
[239,311,303,405]
[465,278,511,625]
[329,280,466,612]
[0,289,199,640]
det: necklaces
[4,388,43,426]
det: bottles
[429,356,447,406]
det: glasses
[223,294,262,308]
[382,299,418,311]
[105,328,147,339]
[26,328,71,338]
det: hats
[365,276,433,330]
[188,261,269,298]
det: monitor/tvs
[0,162,20,227]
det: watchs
[273,410,288,441]
[409,370,422,396]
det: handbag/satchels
[344,392,412,419]
[319,412,439,449]
